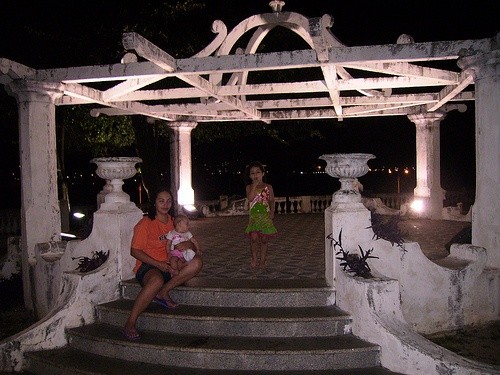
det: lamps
[182,205,200,220]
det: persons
[124,186,203,340]
[244,163,279,269]
[166,214,203,276]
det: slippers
[152,296,179,309]
[120,328,140,342]
